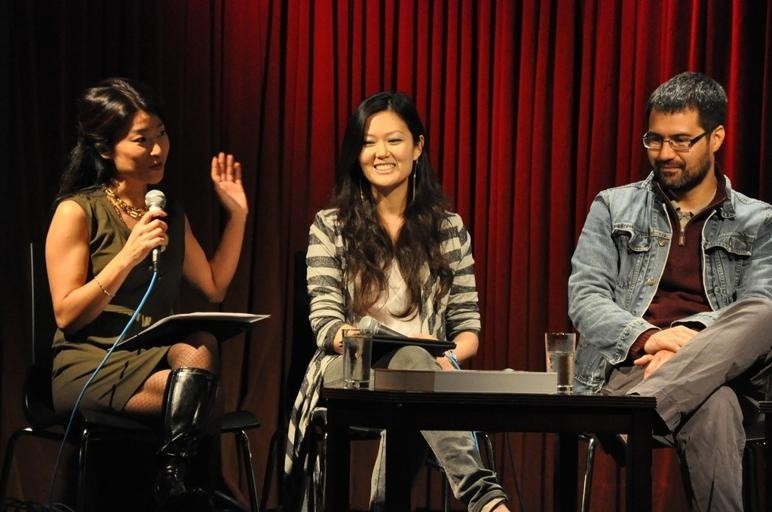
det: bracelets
[95,277,115,299]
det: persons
[284,92,512,512]
[567,71,772,512]
[40,75,249,512]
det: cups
[544,331,578,392]
[342,328,373,386]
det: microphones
[144,188,167,273]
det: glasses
[640,121,723,154]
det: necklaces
[105,189,147,223]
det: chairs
[1,238,262,511]
[264,226,499,510]
[576,380,771,512]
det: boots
[147,364,223,511]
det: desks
[318,377,657,512]
